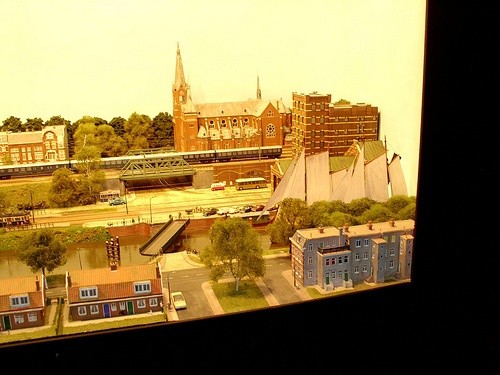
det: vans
[211,183,224,191]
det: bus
[235,178,268,190]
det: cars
[170,292,187,310]
[185,205,265,216]
